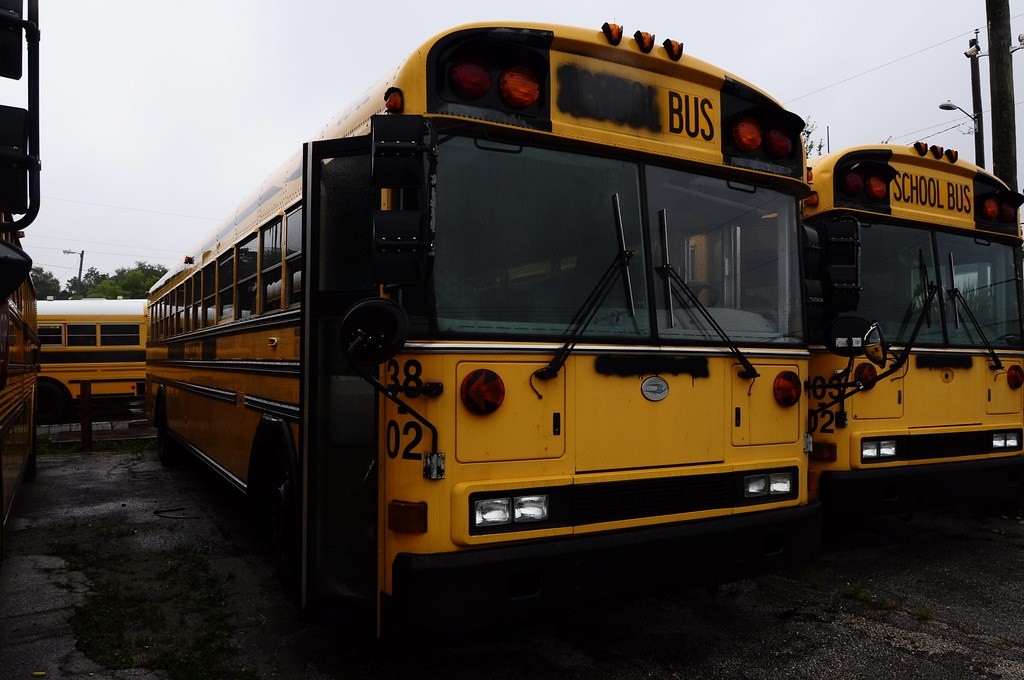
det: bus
[0,0,42,564]
[144,20,811,642]
[33,299,149,422]
[510,140,1023,505]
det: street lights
[939,103,989,311]
[62,248,84,282]
